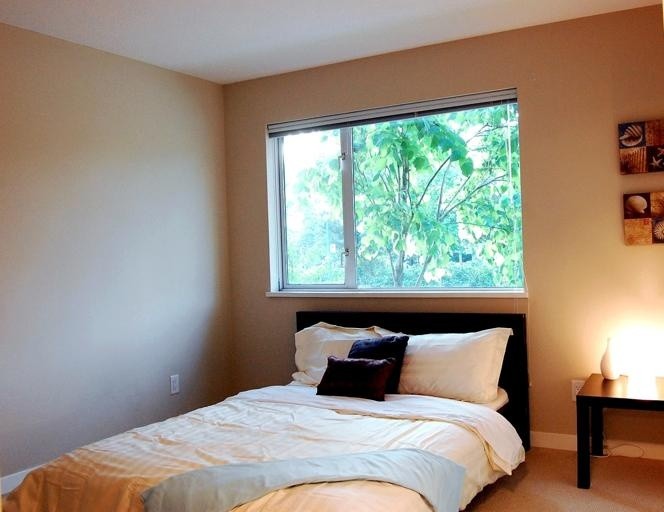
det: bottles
[601,338,619,380]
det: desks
[576,374,664,490]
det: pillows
[290,323,513,404]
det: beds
[37,310,531,512]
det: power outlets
[571,380,585,402]
